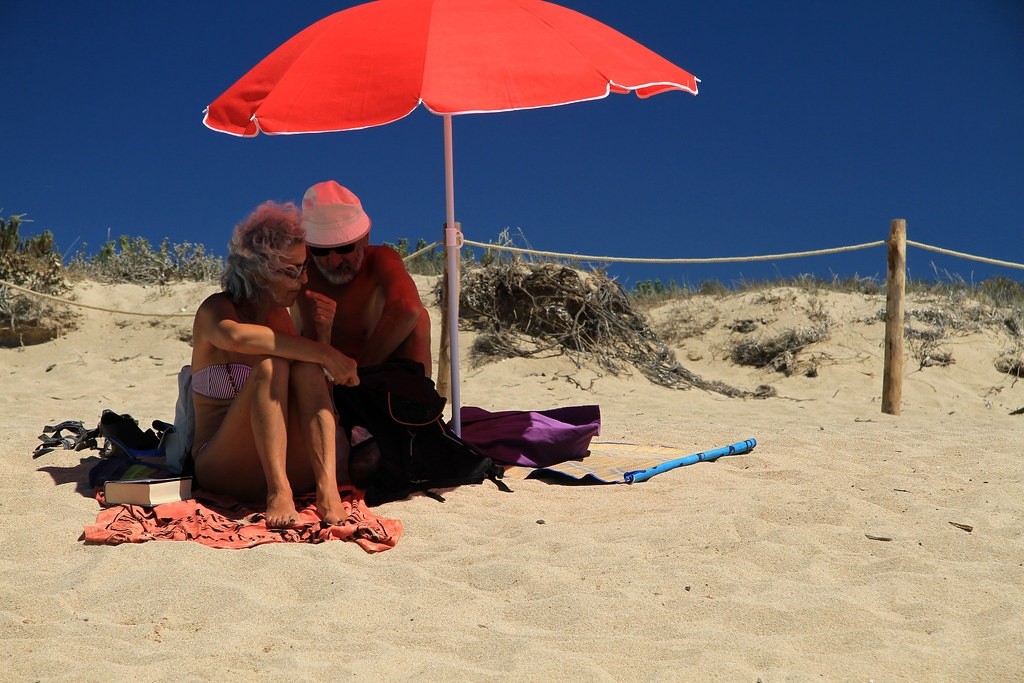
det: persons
[288,179,434,384]
[185,198,362,527]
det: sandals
[32,421,87,458]
[75,411,138,452]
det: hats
[300,180,372,246]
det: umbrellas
[199,0,702,435]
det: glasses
[273,259,309,279]
[309,237,364,257]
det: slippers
[101,411,164,467]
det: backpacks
[369,360,496,490]
